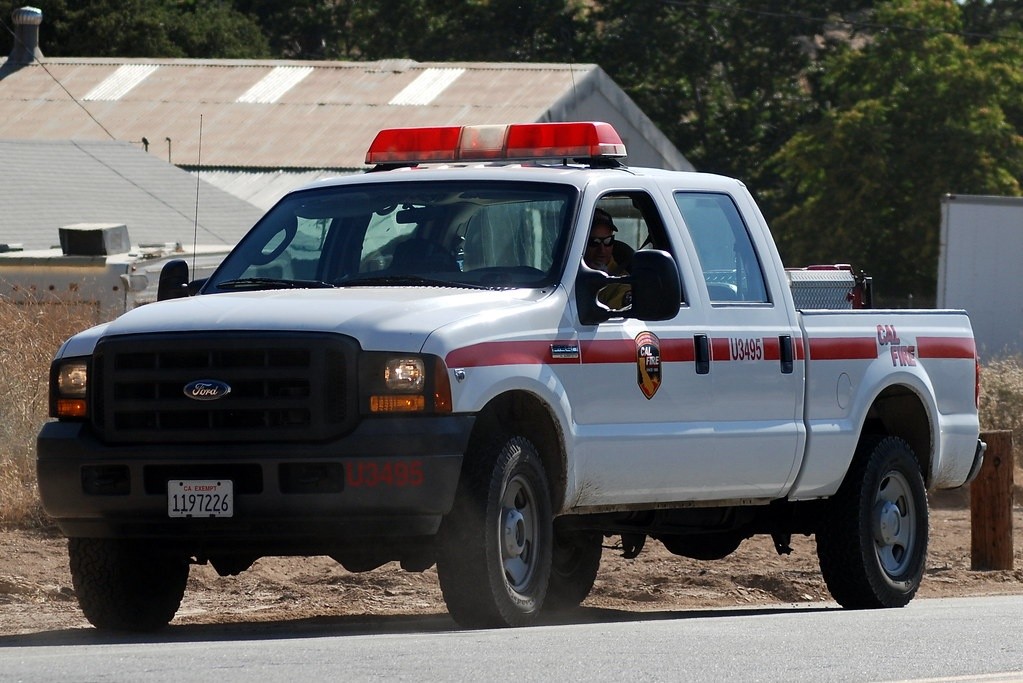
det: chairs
[388,239,462,274]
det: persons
[552,208,633,309]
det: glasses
[587,235,616,248]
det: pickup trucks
[35,121,986,636]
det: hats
[591,208,618,232]
